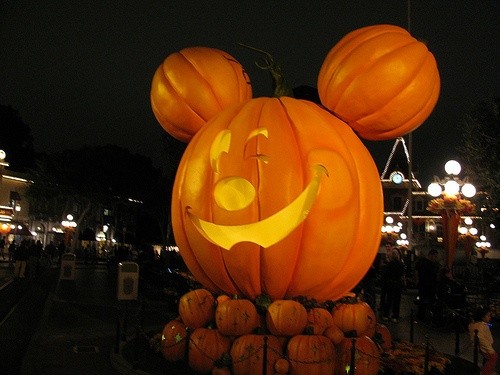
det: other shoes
[392,318,397,322]
[382,317,388,321]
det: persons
[466,304,497,375]
[0,235,191,283]
[363,242,500,323]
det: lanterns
[170,96,384,302]
[286,325,337,375]
[187,322,230,371]
[337,334,380,375]
[150,46,253,143]
[160,315,188,361]
[266,297,331,337]
[331,296,377,339]
[178,288,216,329]
[372,321,392,355]
[230,326,286,375]
[215,293,257,335]
